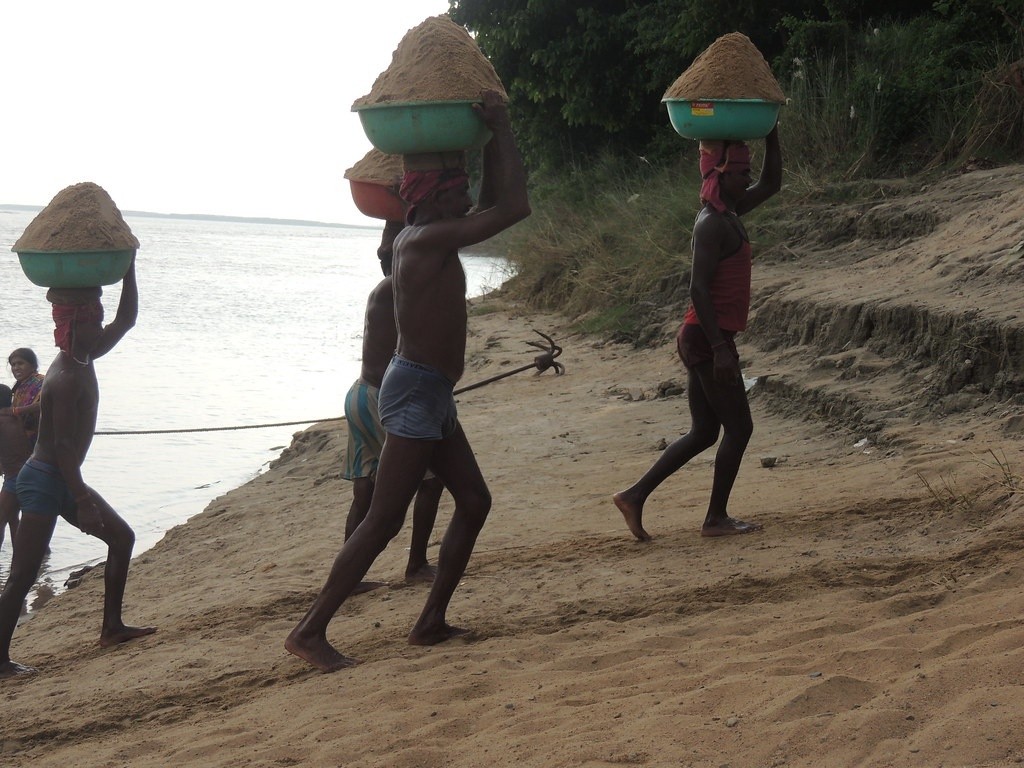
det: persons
[7,348,55,554]
[0,384,32,547]
[0,250,157,679]
[285,88,532,674]
[610,114,781,540]
[339,220,439,595]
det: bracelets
[13,405,19,417]
[713,339,727,351]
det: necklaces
[63,346,92,366]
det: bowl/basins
[352,102,508,154]
[15,247,139,288]
[661,97,790,141]
[343,173,407,222]
